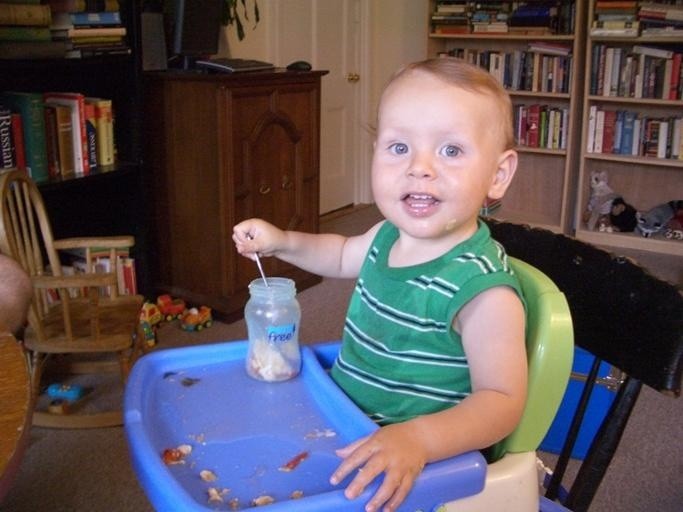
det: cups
[243,275,303,384]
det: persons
[1,255,33,335]
[230,57,526,512]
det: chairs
[1,170,150,430]
[480,215,683,512]
[122,251,574,512]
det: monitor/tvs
[175,0,223,69]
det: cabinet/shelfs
[575,0,683,257]
[159,68,331,325]
[0,0,159,303]
[426,0,586,235]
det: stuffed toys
[586,170,683,241]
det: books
[438,41,572,93]
[42,247,137,305]
[590,0,683,36]
[431,0,575,35]
[591,44,683,100]
[1,1,133,183]
[587,105,683,161]
[513,104,569,149]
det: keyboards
[195,58,274,75]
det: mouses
[287,60,311,72]
